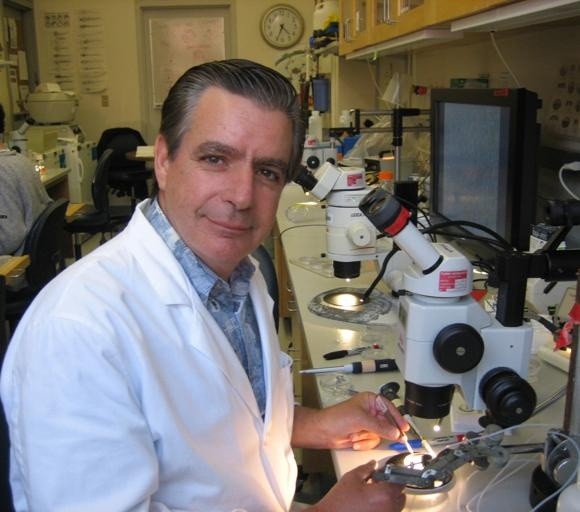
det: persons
[0,99,55,257]
[1,59,409,511]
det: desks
[273,182,569,512]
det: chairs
[99,126,155,222]
[0,195,70,339]
[66,148,114,262]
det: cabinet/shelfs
[337,0,519,55]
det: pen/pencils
[389,434,466,450]
[323,344,379,360]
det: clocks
[259,3,305,49]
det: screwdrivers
[380,383,437,455]
[298,359,398,374]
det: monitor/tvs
[432,86,535,253]
